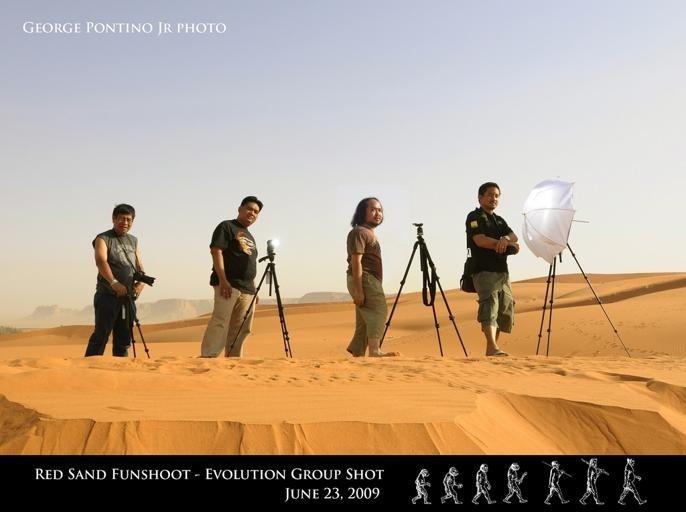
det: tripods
[536,244,632,359]
[128,296,150,359]
[378,222,468,357]
[227,240,292,358]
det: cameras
[133,271,156,287]
[488,246,516,256]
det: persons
[345,196,400,357]
[466,181,519,357]
[201,196,264,358]
[83,203,144,356]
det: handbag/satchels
[461,257,478,292]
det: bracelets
[499,235,511,241]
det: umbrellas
[520,177,589,265]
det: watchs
[110,279,120,287]
